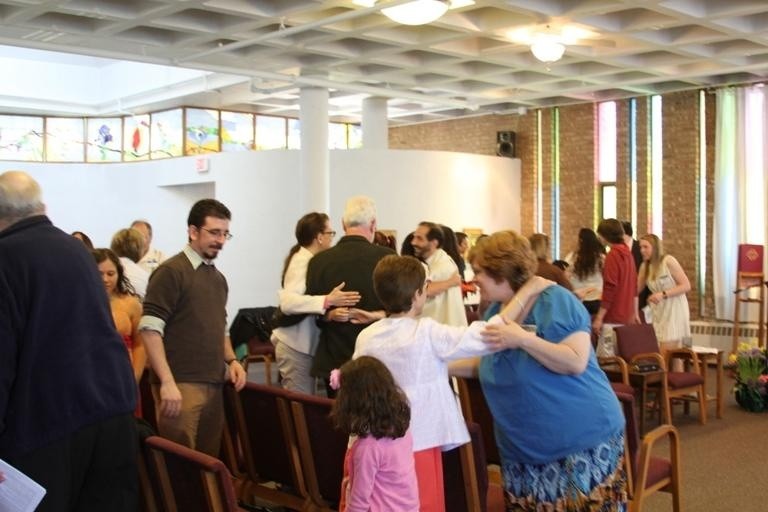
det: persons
[271,196,698,411]
[70,197,248,460]
[0,171,138,512]
[449,231,626,511]
[326,355,420,511]
[339,255,558,511]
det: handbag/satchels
[230,307,278,349]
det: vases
[733,378,768,412]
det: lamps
[347,0,475,25]
[510,21,583,64]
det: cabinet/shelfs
[733,243,768,351]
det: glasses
[199,226,232,240]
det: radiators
[689,321,762,338]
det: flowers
[728,337,768,410]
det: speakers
[496,131,515,158]
